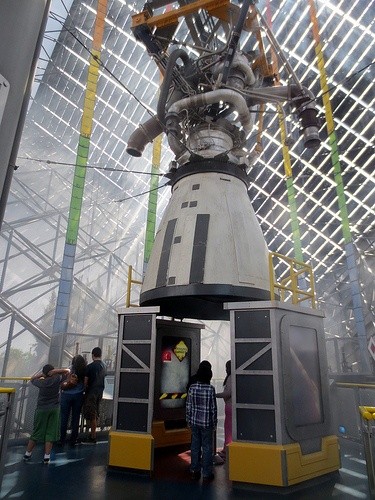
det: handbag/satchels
[60,372,77,390]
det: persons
[23,364,71,464]
[187,360,212,392]
[59,354,86,446]
[80,347,107,445]
[186,367,218,483]
[215,360,232,452]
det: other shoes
[218,452,226,456]
[203,473,215,481]
[194,470,202,478]
[43,458,50,464]
[213,453,224,464]
[84,438,96,443]
[24,454,32,461]
[69,439,78,445]
